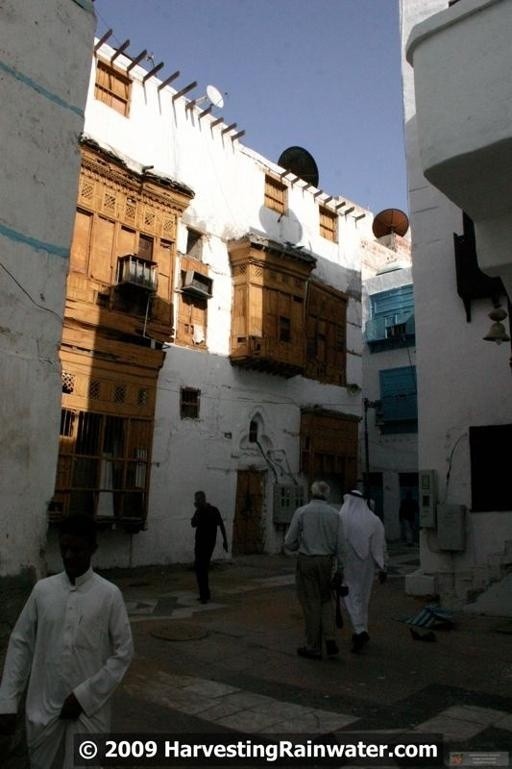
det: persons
[1,514,136,769]
[337,489,388,647]
[190,491,229,603]
[284,479,345,661]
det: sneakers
[351,632,370,650]
[298,641,335,658]
